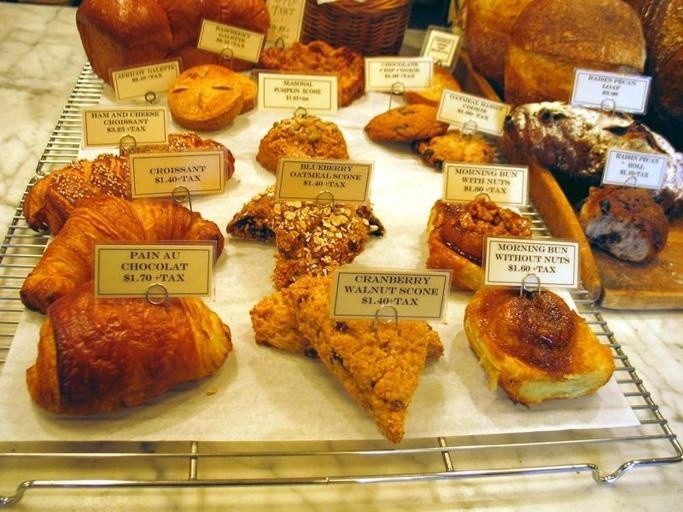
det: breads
[22,0,683,442]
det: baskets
[302,0,412,56]
